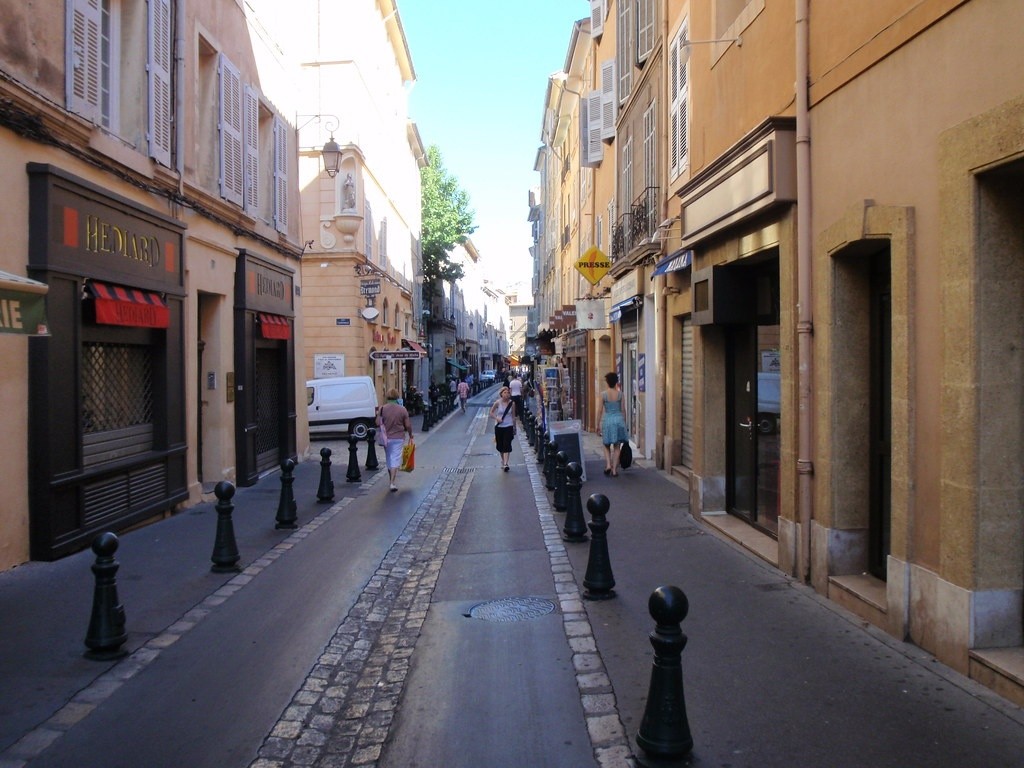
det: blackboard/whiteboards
[553,432,582,477]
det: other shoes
[390,484,398,491]
[502,464,509,472]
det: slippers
[612,471,618,477]
[604,468,611,473]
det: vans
[757,373,782,435]
[306,376,380,440]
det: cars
[479,370,495,382]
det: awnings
[0,270,52,337]
[405,340,429,358]
[447,359,472,371]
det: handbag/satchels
[620,441,632,468]
[402,438,416,472]
[377,407,387,447]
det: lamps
[295,110,343,180]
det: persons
[428,379,440,409]
[445,372,475,412]
[375,388,413,490]
[489,366,525,471]
[595,372,629,477]
